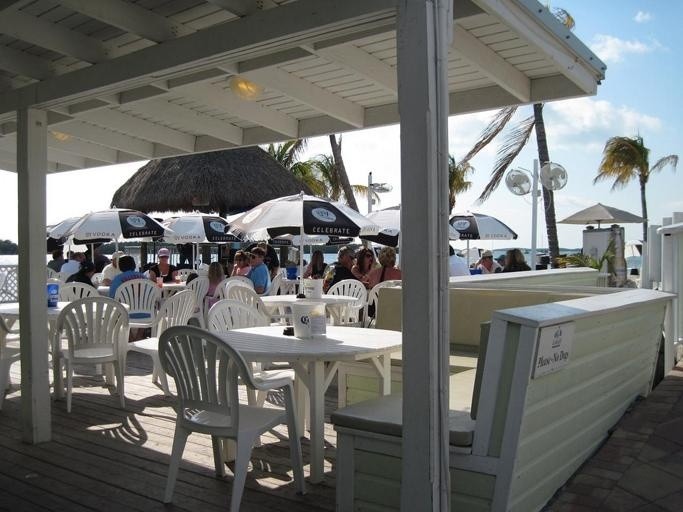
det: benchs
[332,283,675,511]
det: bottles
[173,271,188,284]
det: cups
[156,277,163,288]
[290,302,314,338]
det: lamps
[226,74,265,101]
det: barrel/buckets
[288,300,327,340]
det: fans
[504,167,531,196]
[538,161,567,191]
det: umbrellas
[553,202,648,229]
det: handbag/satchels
[47,282,58,307]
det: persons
[45,242,533,320]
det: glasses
[485,255,493,258]
[365,254,374,258]
[249,253,258,260]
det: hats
[112,251,126,259]
[158,248,170,257]
[338,246,358,256]
[494,255,506,260]
